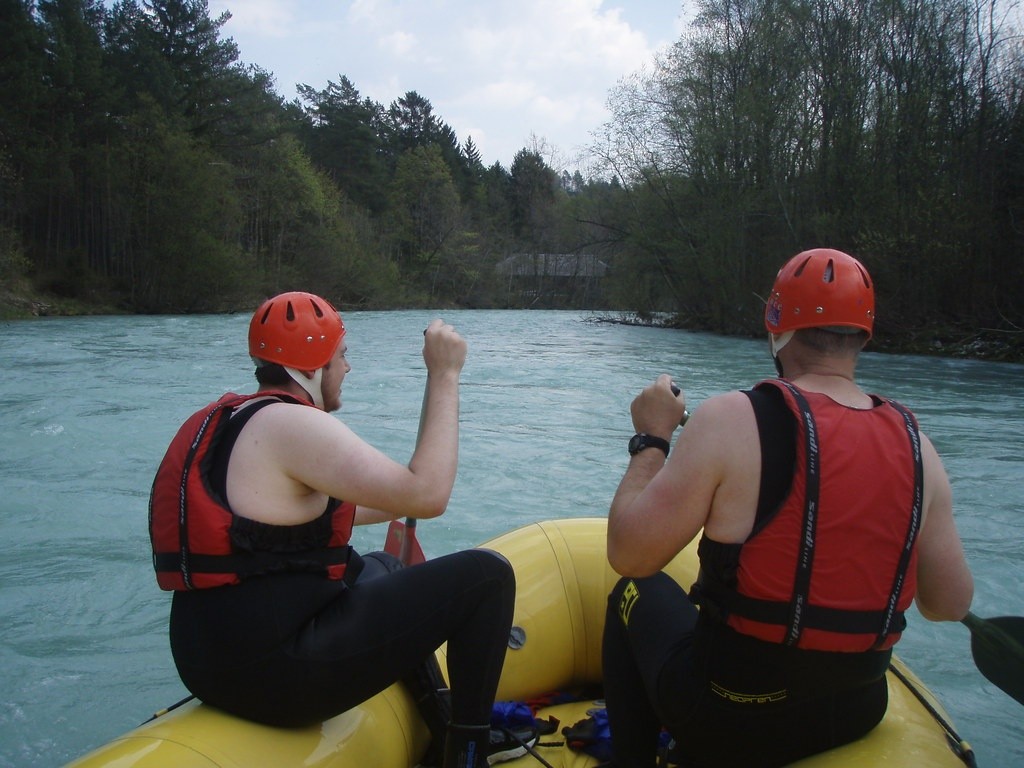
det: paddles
[670,385,1024,704]
[383,516,424,569]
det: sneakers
[476,724,539,762]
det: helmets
[764,248,875,341]
[248,289,345,371]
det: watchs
[628,434,671,457]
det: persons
[599,248,972,768]
[149,292,540,768]
